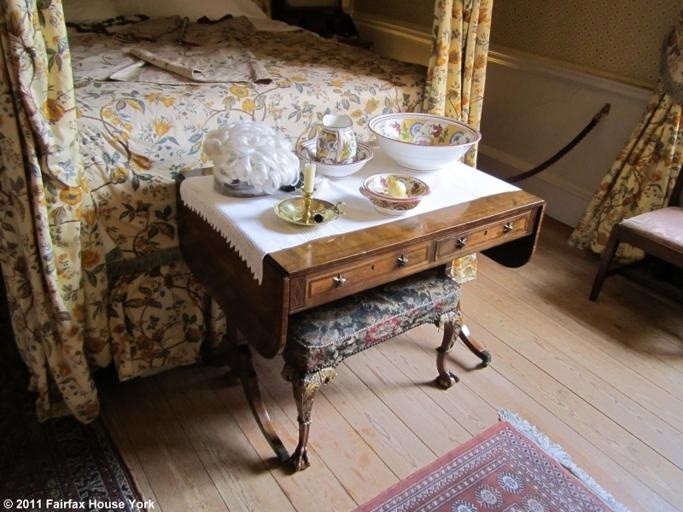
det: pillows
[62,0,266,23]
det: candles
[303,161,318,196]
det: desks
[173,154,547,472]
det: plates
[273,197,337,227]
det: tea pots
[314,112,358,163]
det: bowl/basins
[361,174,432,218]
[365,114,481,171]
[297,140,373,179]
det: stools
[590,202,682,304]
[285,272,467,475]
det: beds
[0,0,488,411]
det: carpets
[2,391,147,512]
[354,410,633,512]
